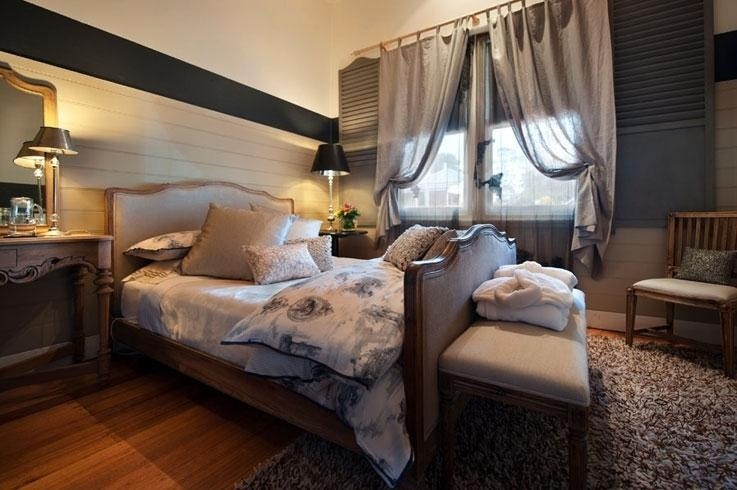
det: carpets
[230,321,736,489]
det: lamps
[28,124,81,238]
[12,141,47,222]
[310,143,351,233]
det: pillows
[674,246,737,284]
[382,224,458,271]
[122,202,333,285]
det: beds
[104,179,520,490]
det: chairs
[624,211,737,378]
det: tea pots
[0,207,12,225]
[6,197,43,235]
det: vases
[338,220,358,232]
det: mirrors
[0,62,61,235]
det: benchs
[437,287,596,489]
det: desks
[322,230,367,257]
[0,234,116,379]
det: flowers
[334,203,361,227]
